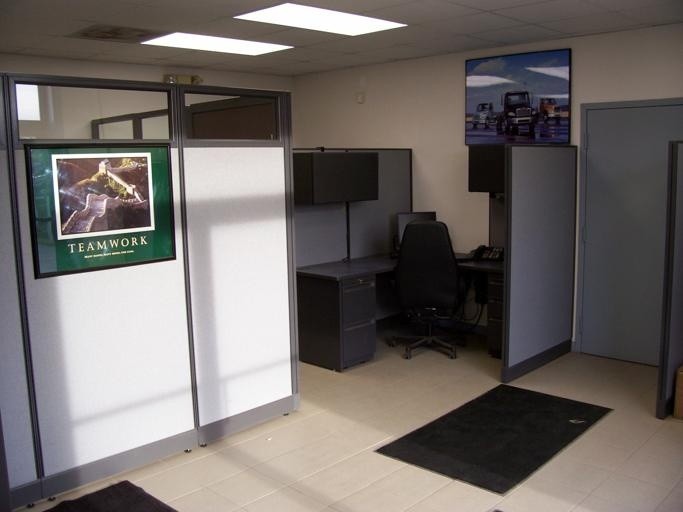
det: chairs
[387,218,473,360]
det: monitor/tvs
[396,212,436,245]
[311,152,379,206]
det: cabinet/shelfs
[292,150,379,205]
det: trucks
[471,102,502,130]
[497,90,537,131]
[534,97,561,124]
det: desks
[296,251,504,374]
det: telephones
[473,245,504,261]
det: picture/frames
[463,47,572,146]
[21,142,176,281]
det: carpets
[371,383,617,496]
[41,479,179,511]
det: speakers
[468,144,505,193]
